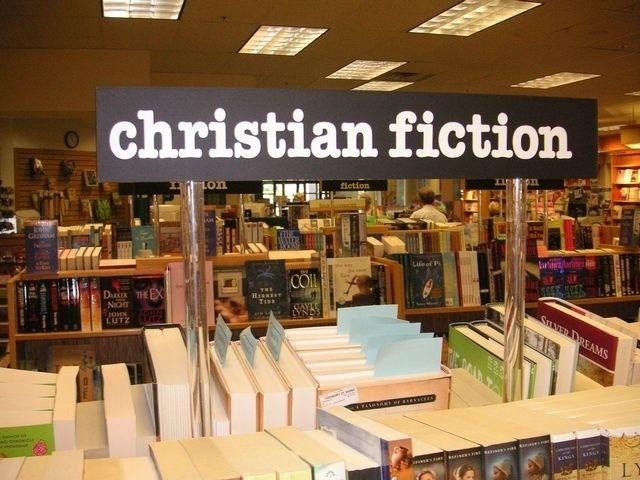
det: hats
[488,200,502,214]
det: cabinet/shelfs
[439,179,491,222]
[400,263,507,321]
[525,248,640,309]
[610,151,640,228]
[7,257,186,369]
[567,135,611,219]
[205,252,398,333]
[493,188,566,224]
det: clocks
[64,131,80,149]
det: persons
[360,193,380,216]
[410,188,448,223]
[344,274,371,306]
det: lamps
[620,103,640,151]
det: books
[388,250,503,310]
[0,448,151,480]
[0,343,154,457]
[265,227,324,252]
[15,275,166,335]
[245,259,288,321]
[270,249,271,249]
[167,260,216,328]
[450,295,640,409]
[131,225,160,258]
[235,241,272,255]
[494,221,559,263]
[23,219,59,276]
[288,266,323,320]
[525,254,640,302]
[117,240,132,259]
[59,222,113,260]
[367,227,465,258]
[619,186,640,202]
[619,203,639,245]
[614,168,639,185]
[243,221,265,249]
[60,244,103,270]
[324,211,367,256]
[151,203,243,253]
[327,256,374,314]
[560,216,619,250]
[151,423,343,480]
[371,260,394,305]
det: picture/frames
[83,168,98,188]
